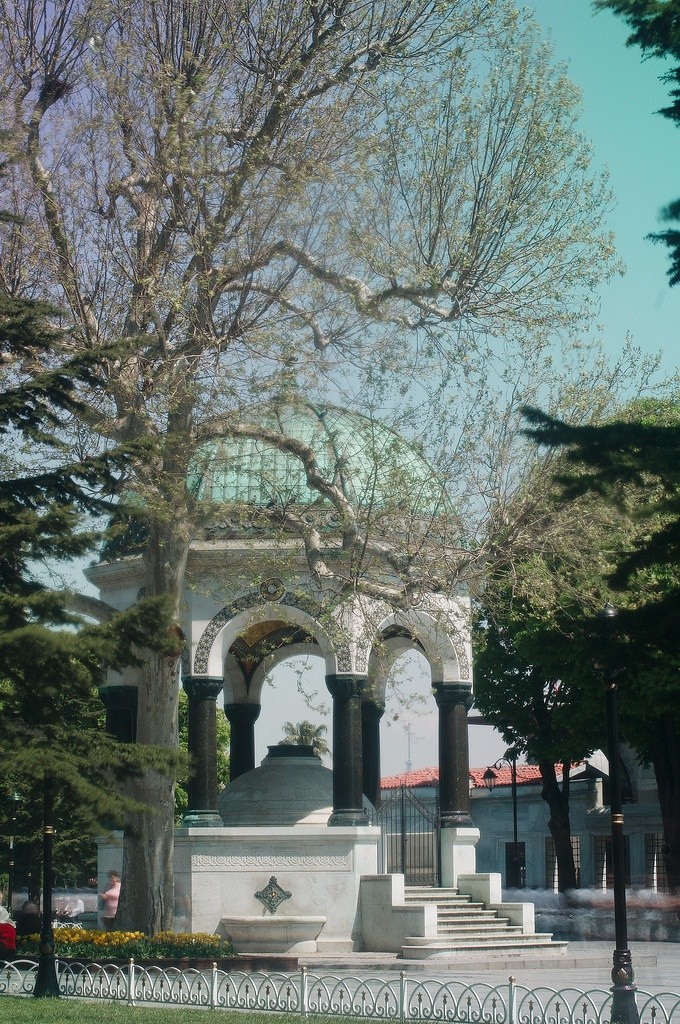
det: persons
[57,896,84,918]
[98,870,121,931]
[0,905,17,969]
